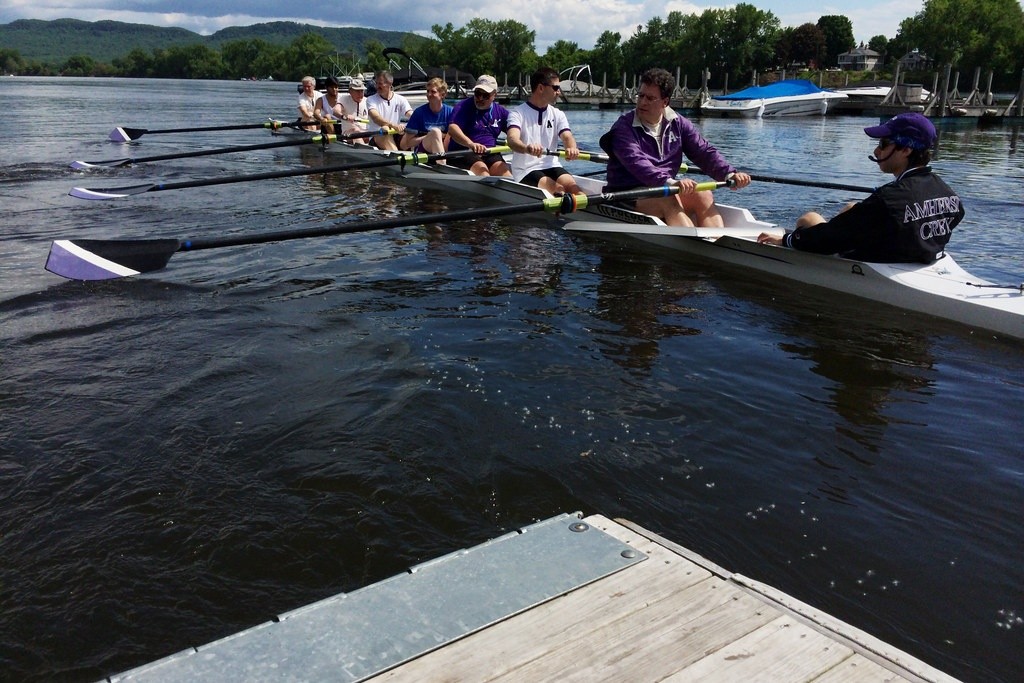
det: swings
[108,118,341,142]
[353,118,372,122]
[69,128,395,172]
[542,145,874,192]
[67,144,513,198]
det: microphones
[868,147,896,163]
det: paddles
[47,176,736,279]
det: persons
[314,76,342,134]
[366,71,413,151]
[400,78,453,166]
[507,66,586,198]
[298,76,323,131]
[448,75,514,178]
[757,113,965,265]
[599,69,752,228]
[333,79,372,146]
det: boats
[701,78,849,119]
[297,71,374,93]
[834,82,941,108]
[383,45,639,122]
[267,117,1024,346]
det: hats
[473,75,497,93]
[349,79,367,90]
[864,113,937,143]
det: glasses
[878,140,900,150]
[475,91,491,100]
[543,84,560,91]
[637,92,666,103]
